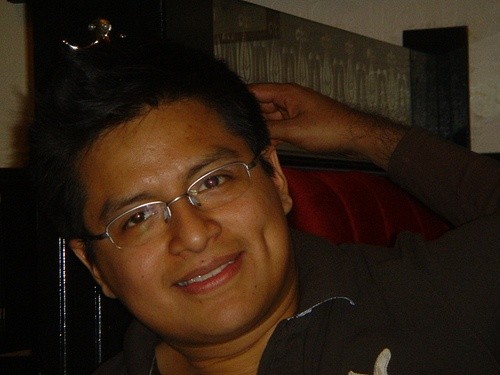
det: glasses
[74,140,276,251]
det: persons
[25,43,500,375]
